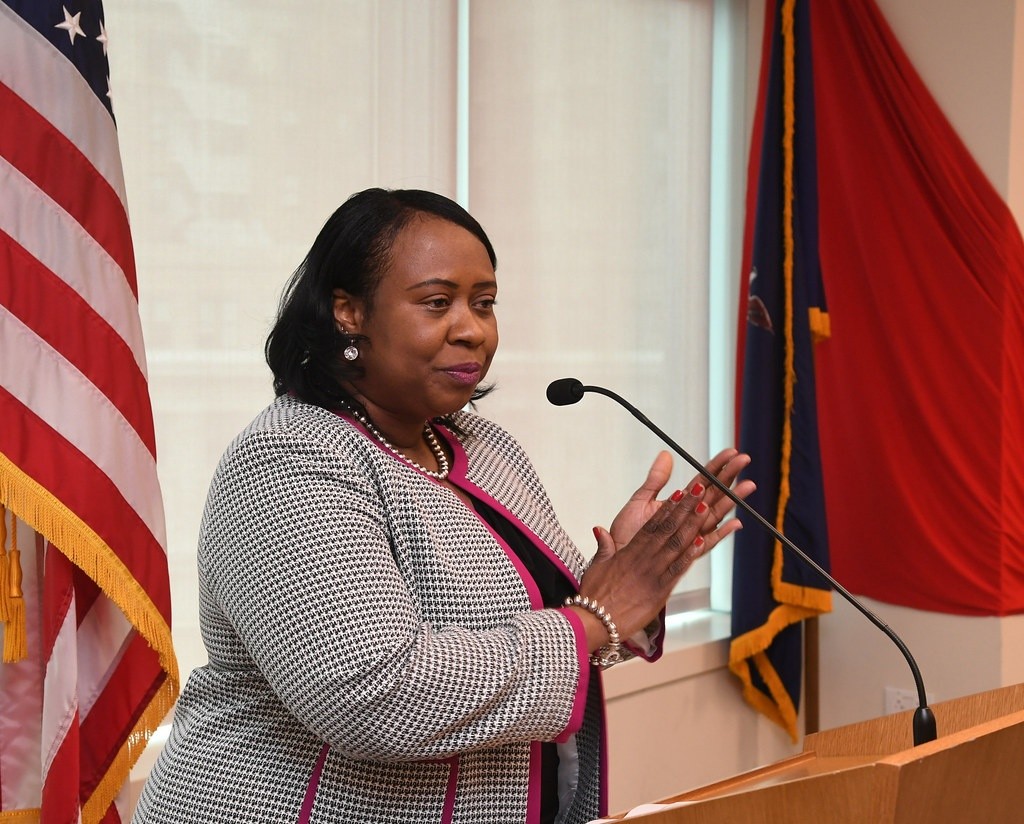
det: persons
[140,186,756,824]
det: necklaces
[338,397,450,482]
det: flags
[3,0,185,823]
[736,0,1024,616]
[727,0,835,741]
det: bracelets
[558,595,619,667]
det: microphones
[547,378,936,749]
